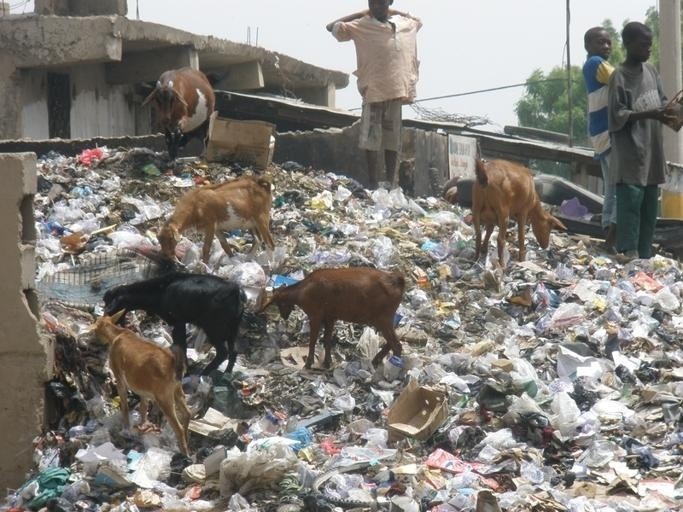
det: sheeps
[258,266,408,370]
[100,271,245,374]
[154,175,275,265]
[471,157,569,270]
[81,306,192,458]
[139,65,217,163]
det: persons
[326,0,423,190]
[399,159,412,196]
[608,23,682,258]
[585,27,616,252]
[429,163,441,197]
